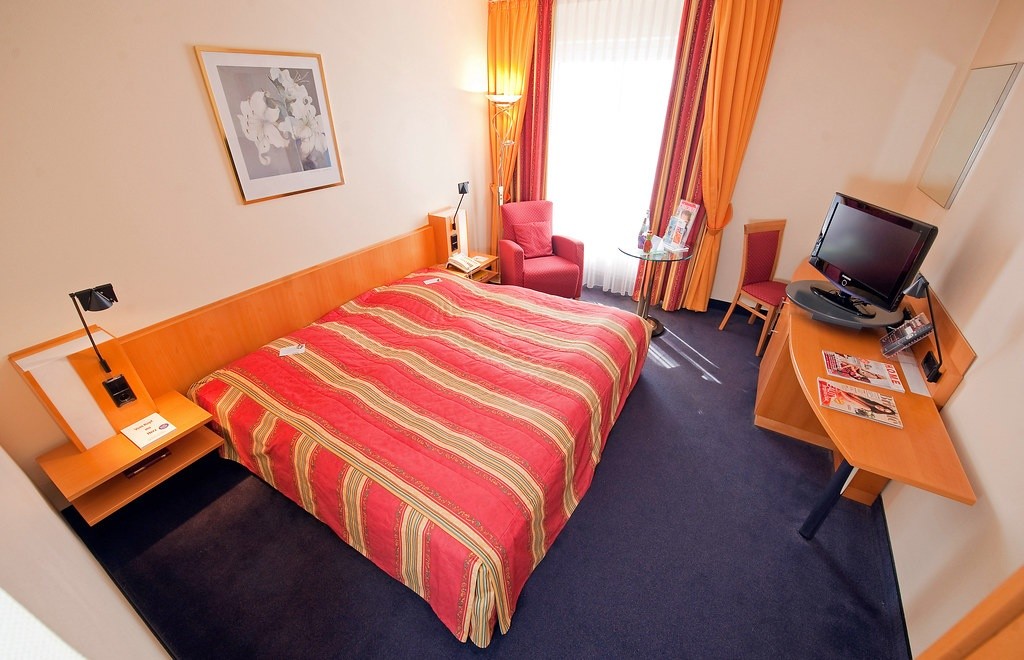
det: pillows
[512,220,555,259]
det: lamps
[451,181,470,231]
[485,93,522,284]
[901,272,944,382]
[69,281,118,373]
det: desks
[751,257,978,540]
[618,238,696,337]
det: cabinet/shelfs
[427,206,499,283]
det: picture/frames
[194,43,346,207]
[916,62,1024,209]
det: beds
[118,224,655,650]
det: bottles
[638,210,650,248]
[644,232,652,252]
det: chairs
[719,220,788,357]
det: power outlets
[920,350,938,377]
[102,374,137,408]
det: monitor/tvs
[809,192,938,320]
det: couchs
[497,200,584,301]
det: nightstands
[8,324,226,528]
